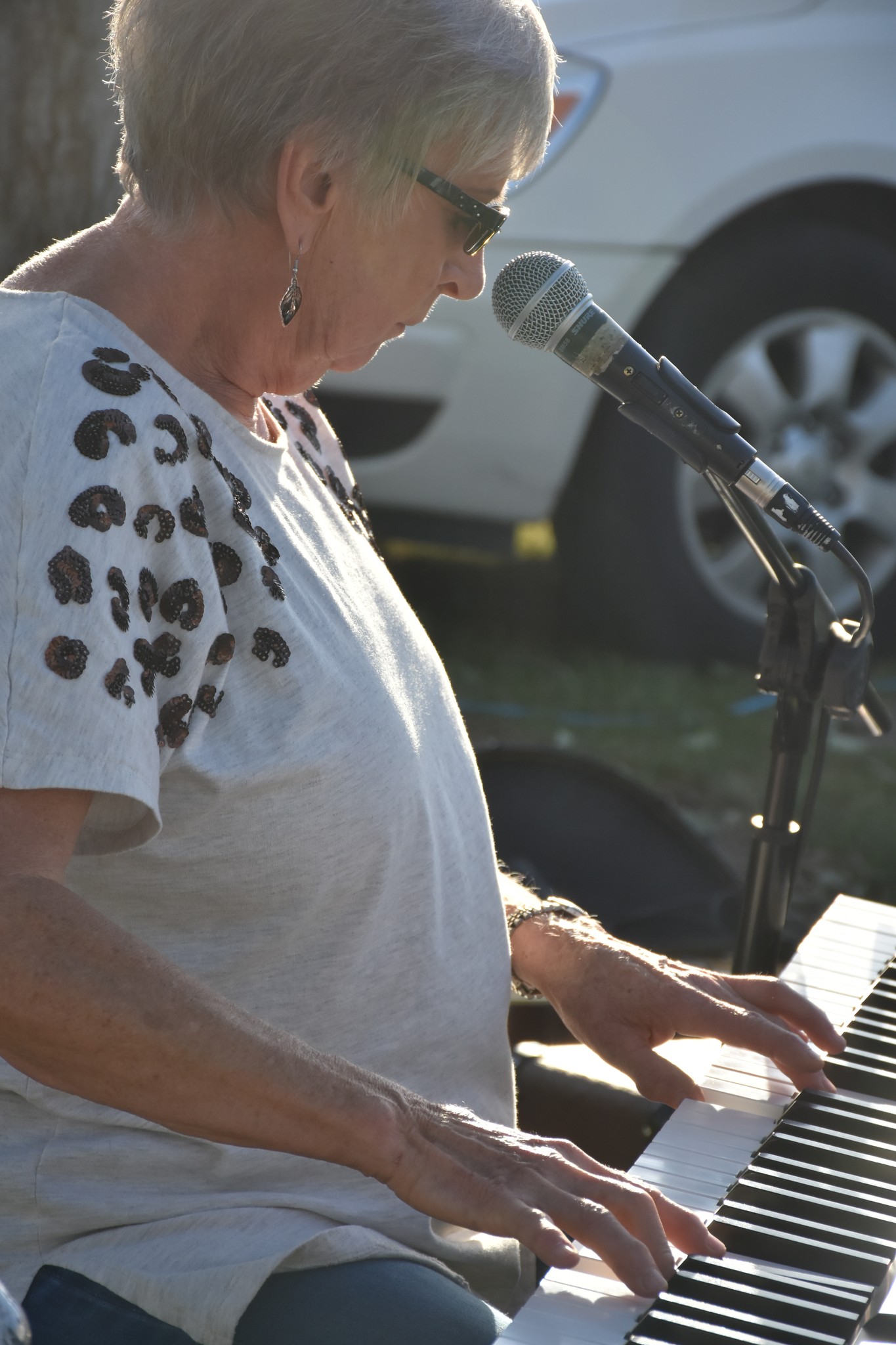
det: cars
[310,0,896,680]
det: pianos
[494,890,896,1345]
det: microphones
[488,250,843,553]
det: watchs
[506,897,589,1001]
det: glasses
[390,153,512,258]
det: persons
[0,0,845,1345]
